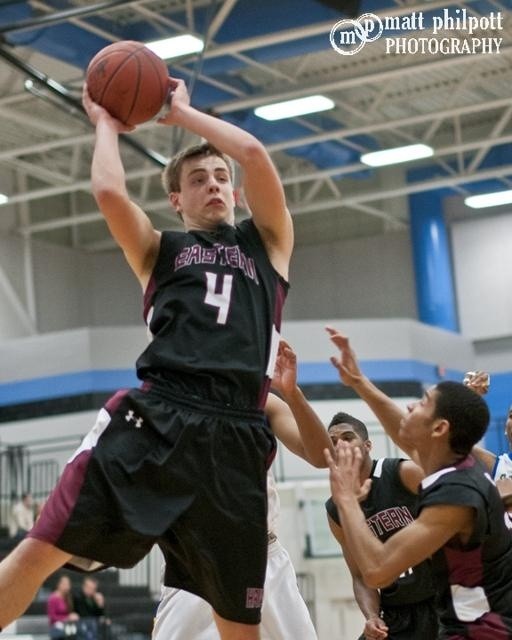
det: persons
[0,77,294,640]
[459,367,511,514]
[323,327,511,639]
[8,488,114,640]
[326,412,458,640]
[149,338,336,640]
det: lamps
[252,94,335,122]
[465,190,511,211]
[359,143,436,168]
[144,30,205,61]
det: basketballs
[86,40,169,126]
